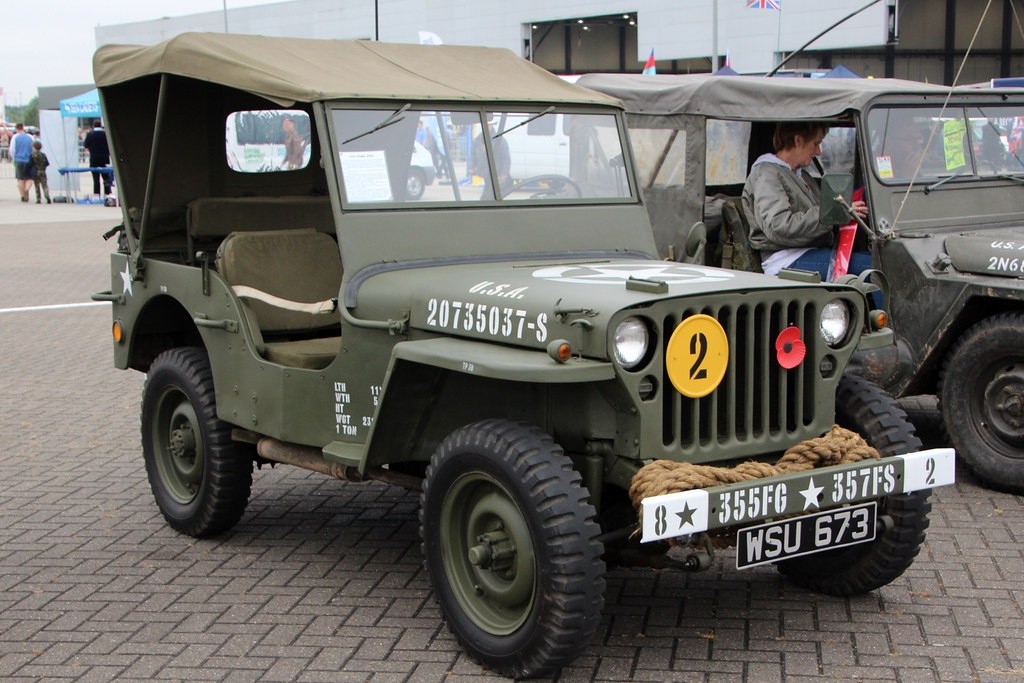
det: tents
[60,88,103,202]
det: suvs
[92,30,958,679]
[574,72,1023,493]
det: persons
[9,119,114,200]
[413,119,513,200]
[742,121,887,311]
[281,116,304,168]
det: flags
[745,1,781,11]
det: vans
[486,75,575,182]
[225,111,436,205]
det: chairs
[214,228,348,371]
[722,196,764,274]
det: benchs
[188,193,336,264]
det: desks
[58,166,115,206]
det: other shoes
[21,197,24,202]
[23,191,29,203]
